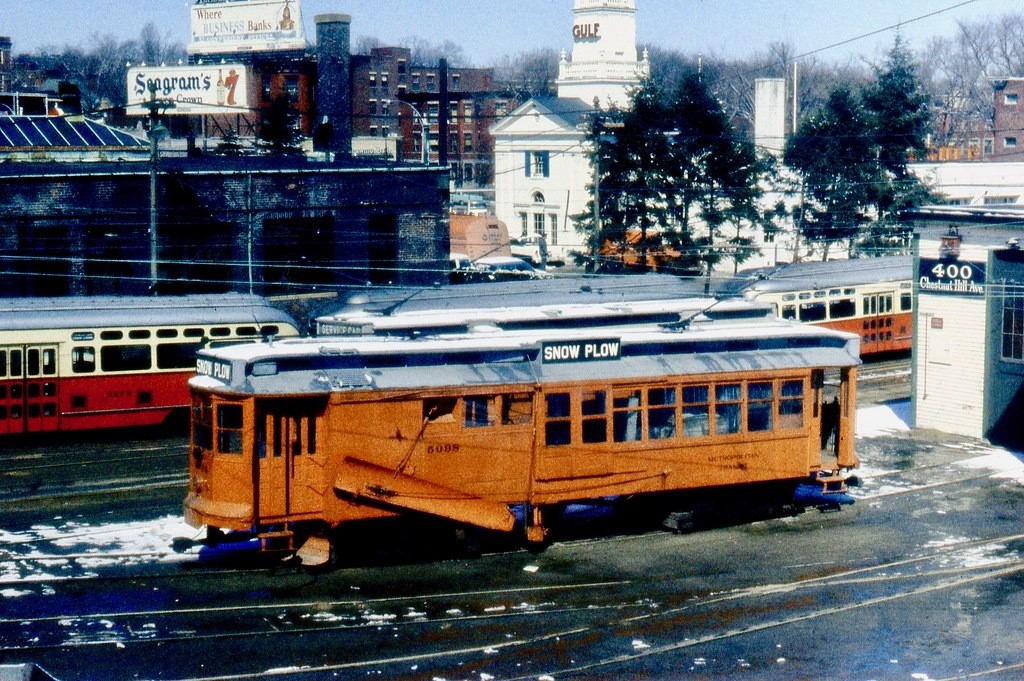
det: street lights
[386,99,425,164]
[697,52,703,83]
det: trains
[0,255,916,445]
[181,321,862,567]
